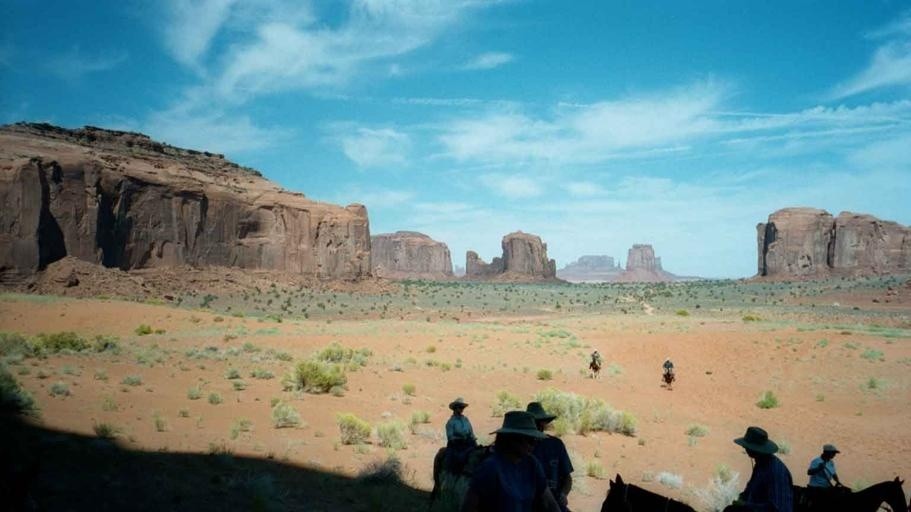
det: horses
[591,358,601,380]
[600,473,697,512]
[663,368,673,390]
[793,476,908,512]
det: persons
[662,357,675,382]
[807,444,844,487]
[722,427,793,512]
[589,350,602,369]
[445,397,573,512]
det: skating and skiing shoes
[449,397,469,410]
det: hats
[489,402,557,440]
[823,444,840,453]
[733,426,779,454]
